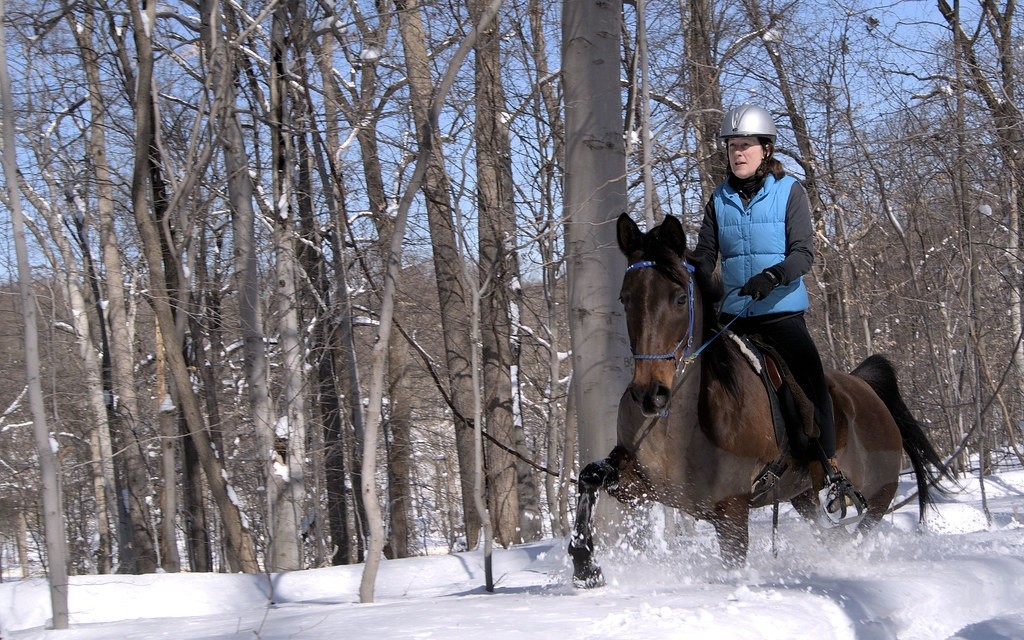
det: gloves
[738,272,775,303]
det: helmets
[719,105,776,147]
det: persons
[693,104,838,474]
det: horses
[565,210,970,590]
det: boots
[811,456,858,514]
